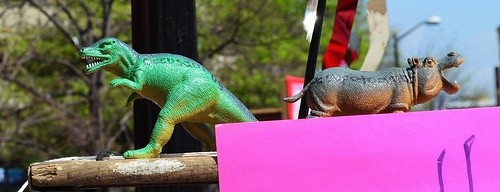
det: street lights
[393,16,442,70]
